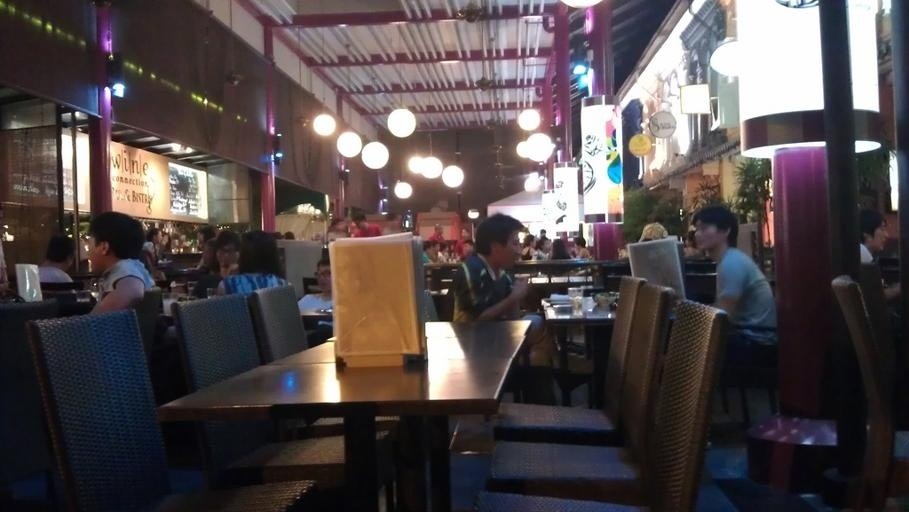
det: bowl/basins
[590,292,616,308]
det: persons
[143,227,295,299]
[88,212,156,314]
[641,204,778,361]
[520,229,594,275]
[453,213,556,405]
[312,212,382,293]
[1,236,77,293]
[860,211,901,297]
[423,224,475,288]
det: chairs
[829,277,907,510]
[496,275,647,442]
[486,283,673,490]
[102,288,165,378]
[251,284,400,437]
[477,299,724,509]
[26,307,316,510]
[171,294,392,511]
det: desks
[159,318,532,510]
[538,294,618,405]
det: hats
[638,223,668,241]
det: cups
[567,286,584,310]
[15,264,43,302]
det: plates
[552,303,572,311]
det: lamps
[628,135,653,154]
[107,51,125,97]
[649,110,679,139]
[678,84,723,134]
[710,36,752,78]
